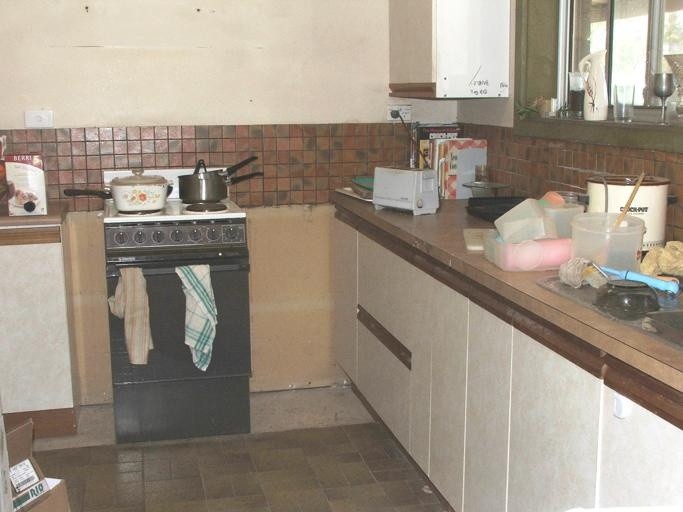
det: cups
[613,85,635,123]
[495,190,583,272]
[548,71,587,119]
[473,165,489,185]
[573,211,651,273]
[472,188,495,198]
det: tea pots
[576,49,610,123]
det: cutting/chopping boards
[462,226,494,252]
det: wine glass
[652,71,677,129]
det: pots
[64,167,172,213]
[576,173,678,257]
[178,155,258,204]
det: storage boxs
[6,417,71,512]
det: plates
[334,176,373,204]
[463,181,509,190]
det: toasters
[372,164,440,217]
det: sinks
[646,310,682,333]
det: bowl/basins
[592,279,664,315]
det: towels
[173,264,219,372]
[107,267,155,367]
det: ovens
[103,255,249,445]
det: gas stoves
[103,197,246,253]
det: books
[462,226,499,252]
[409,123,488,199]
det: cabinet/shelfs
[462,274,682,512]
[0,202,86,443]
[356,212,469,512]
[387,0,509,101]
[325,195,359,393]
